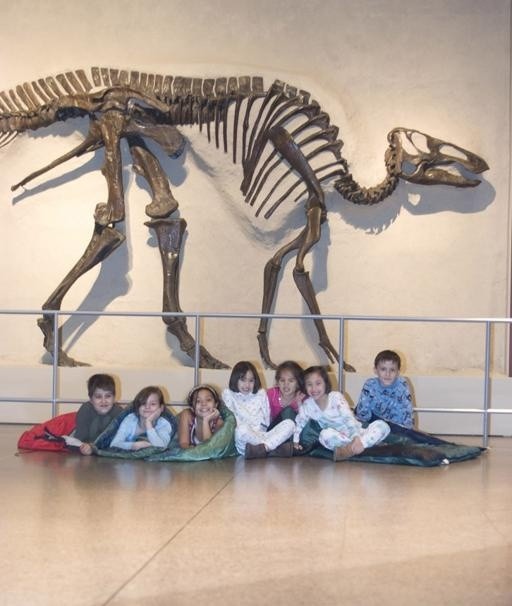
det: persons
[178,383,224,449]
[75,375,122,455]
[355,350,415,431]
[293,365,391,462]
[268,360,306,423]
[222,361,296,456]
[109,386,172,451]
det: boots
[269,441,293,458]
[334,445,354,463]
[245,443,268,459]
